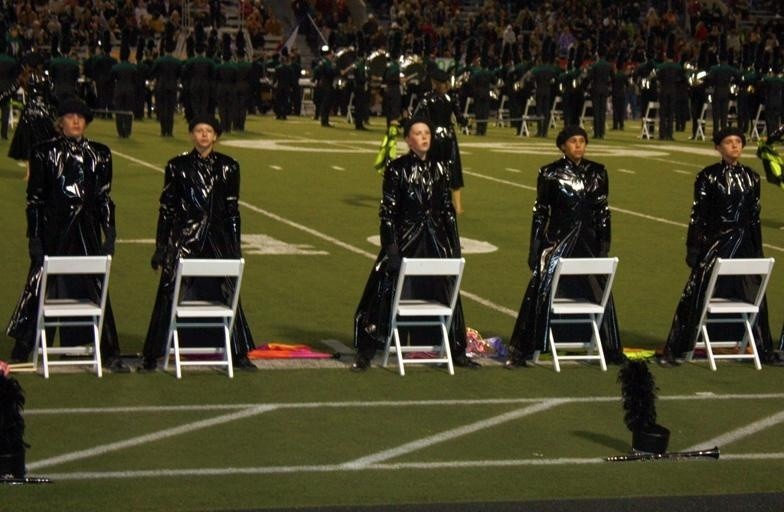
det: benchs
[41,6,782,61]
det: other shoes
[514,129,549,138]
[101,355,130,373]
[512,347,533,366]
[141,351,157,372]
[232,352,258,372]
[354,122,368,132]
[321,122,332,128]
[461,126,487,136]
[605,351,632,367]
[221,124,244,133]
[11,340,36,364]
[119,134,130,139]
[758,351,784,367]
[671,350,687,363]
[160,132,174,138]
[452,353,483,369]
[592,124,625,139]
[352,348,375,371]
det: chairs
[0,62,784,137]
[31,254,112,378]
[380,257,466,376]
[160,256,246,380]
[533,256,619,372]
[686,257,775,371]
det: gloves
[527,247,538,272]
[150,247,168,270]
[385,253,401,276]
[99,236,115,258]
[29,236,45,268]
[685,253,698,269]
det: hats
[630,422,670,453]
[0,33,784,85]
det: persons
[660,126,773,365]
[509,125,630,366]
[142,114,259,373]
[399,70,472,215]
[0,52,61,182]
[351,117,472,372]
[5,98,131,373]
